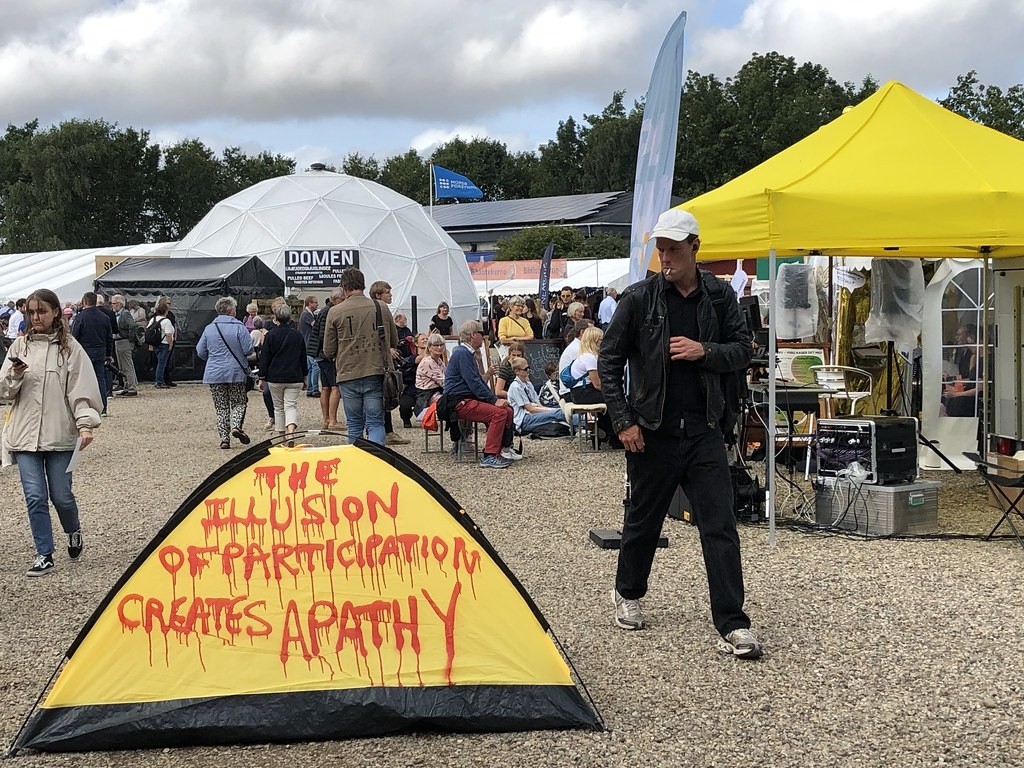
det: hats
[648,209,699,242]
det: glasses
[473,330,485,337]
[518,366,530,371]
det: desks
[737,381,840,498]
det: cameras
[104,358,127,382]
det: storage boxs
[811,476,942,535]
[986,450,1024,515]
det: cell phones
[8,357,27,367]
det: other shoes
[287,434,295,448]
[403,420,413,429]
[385,432,412,445]
[101,411,107,417]
[116,391,137,398]
[307,391,321,398]
[155,382,177,389]
[322,421,347,431]
[220,441,230,449]
[265,418,274,429]
[230,427,251,445]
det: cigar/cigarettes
[665,267,671,276]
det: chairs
[804,365,877,482]
[962,452,1024,548]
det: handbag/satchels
[702,270,749,449]
[246,376,255,391]
[384,370,403,411]
[531,423,576,440]
[560,366,578,389]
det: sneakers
[27,554,54,577]
[67,526,84,560]
[480,453,514,469]
[500,448,522,460]
[610,586,648,630]
[717,629,764,658]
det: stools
[564,402,607,453]
[421,408,450,453]
[450,419,484,464]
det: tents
[667,80,1024,546]
[4,430,611,758]
[90,252,286,380]
[0,241,180,315]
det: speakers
[781,265,810,309]
[871,258,911,313]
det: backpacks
[144,316,166,347]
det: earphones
[693,243,697,250]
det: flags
[431,162,484,200]
[627,10,687,286]
[539,241,555,310]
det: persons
[594,204,764,661]
[257,304,310,448]
[323,267,400,449]
[195,296,254,449]
[443,320,513,468]
[0,284,993,462]
[0,288,105,579]
